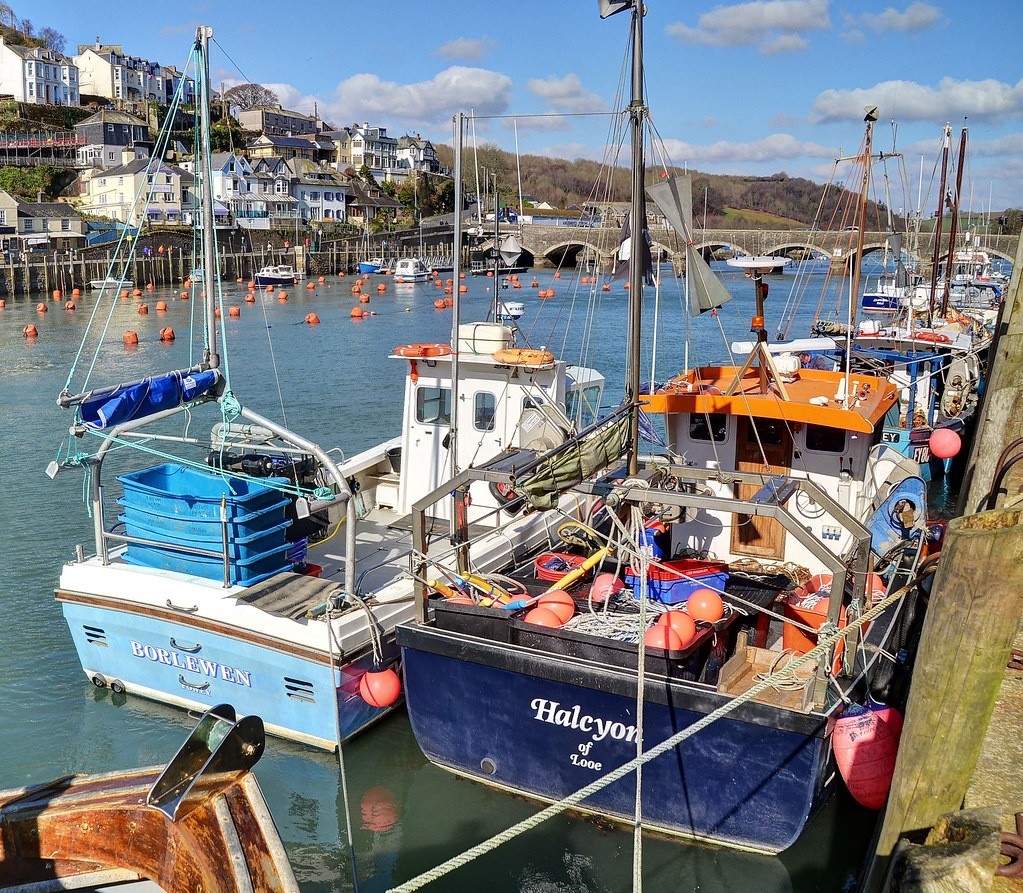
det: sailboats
[44,20,651,751]
[356,204,394,275]
[396,0,1004,860]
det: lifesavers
[915,332,949,342]
[396,344,450,356]
[656,383,721,396]
[494,349,555,364]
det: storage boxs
[423,559,740,687]
[781,574,881,674]
[114,463,324,588]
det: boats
[179,267,219,283]
[392,212,433,283]
[87,274,134,289]
[295,273,306,280]
[254,262,295,289]
[470,255,528,275]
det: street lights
[480,166,485,211]
[415,177,421,220]
[491,174,496,212]
[291,209,298,246]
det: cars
[808,228,822,231]
[793,228,808,231]
[842,226,859,231]
